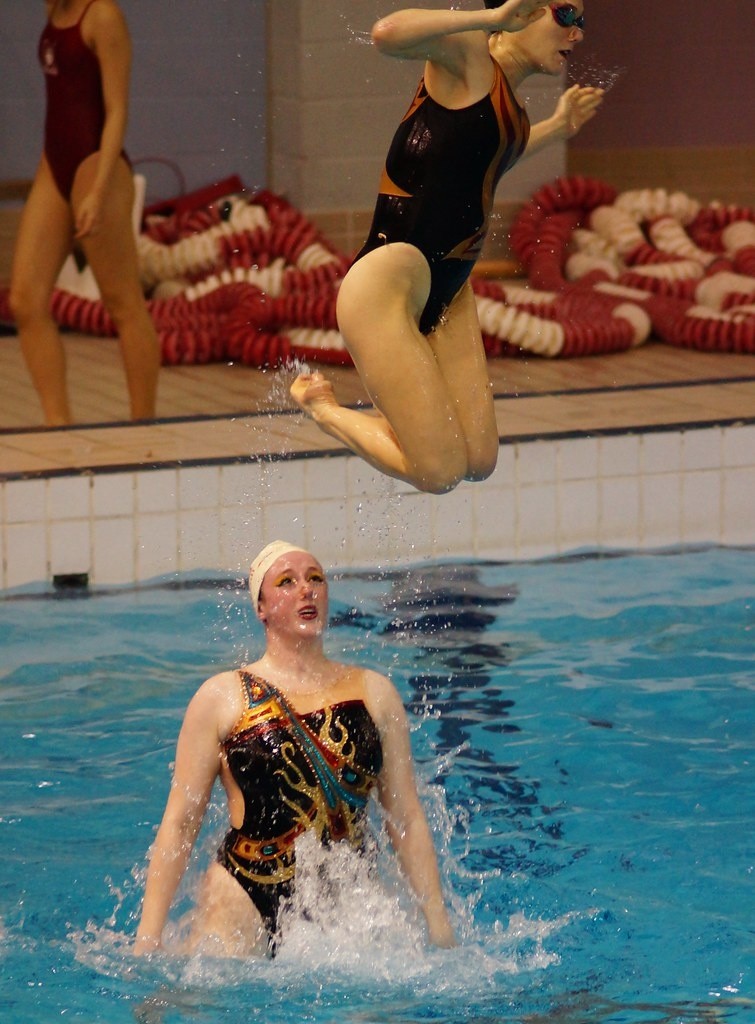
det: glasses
[549,3,587,32]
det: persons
[134,539,453,1023]
[10,0,162,427]
[290,0,605,494]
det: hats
[249,541,311,626]
